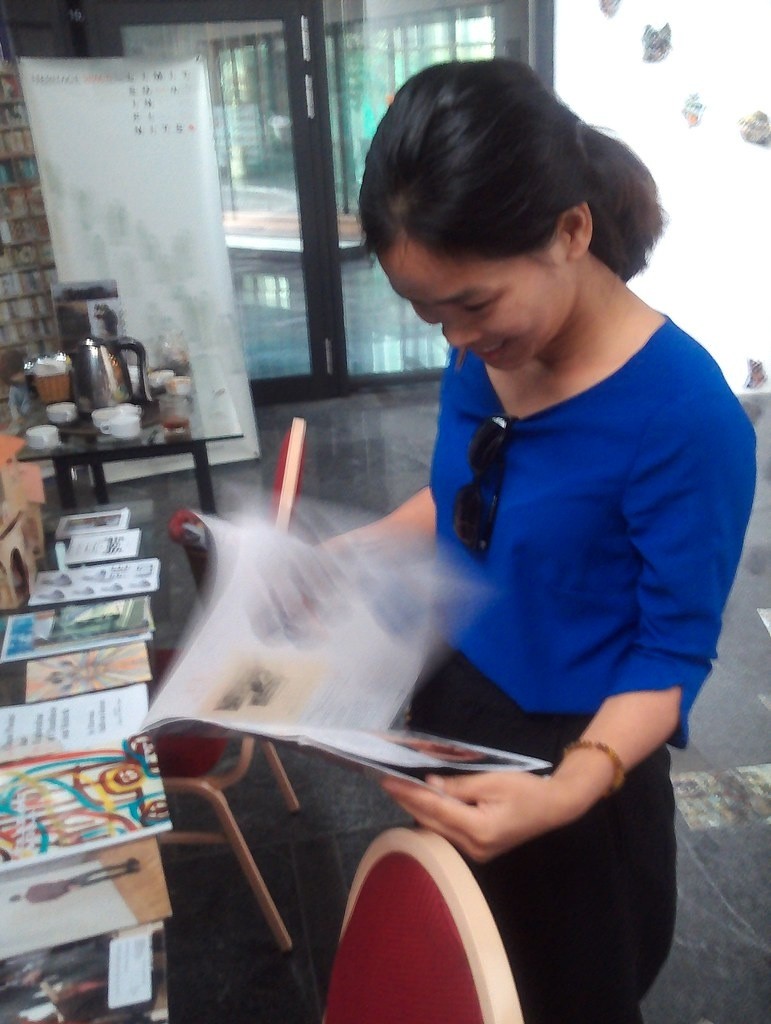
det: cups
[27,423,60,450]
[47,401,78,424]
[126,364,196,427]
[91,402,142,438]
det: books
[1,918,170,1024]
[0,73,64,433]
[64,528,142,568]
[135,477,553,810]
[54,507,134,540]
[1,738,175,873]
[1,834,175,960]
[24,638,154,704]
[27,557,162,607]
[1,594,157,664]
[1,682,150,767]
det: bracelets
[563,741,628,798]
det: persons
[297,56,762,1023]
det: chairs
[149,417,310,957]
[322,826,525,1024]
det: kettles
[73,333,153,414]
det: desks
[0,610,168,1023]
[6,349,246,519]
